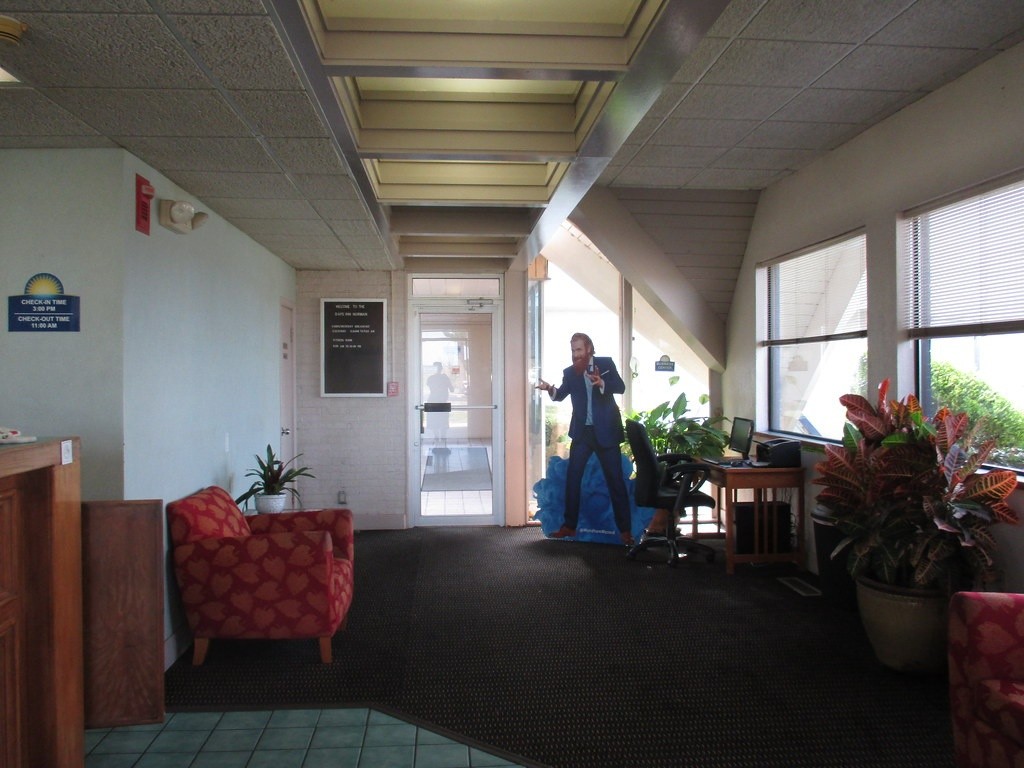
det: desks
[691,455,808,575]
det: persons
[534,332,635,547]
[425,361,454,449]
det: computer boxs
[731,501,791,568]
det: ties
[586,365,593,374]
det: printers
[751,437,801,468]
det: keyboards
[701,457,731,465]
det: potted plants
[556,376,732,534]
[235,444,317,519]
[810,377,1023,673]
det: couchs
[948,592,1024,768]
[167,485,354,666]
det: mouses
[731,460,743,467]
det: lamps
[160,201,209,235]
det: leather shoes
[621,532,635,545]
[550,526,576,538]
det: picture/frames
[320,298,387,397]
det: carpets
[164,525,957,768]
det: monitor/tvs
[729,417,754,459]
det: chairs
[625,419,717,568]
